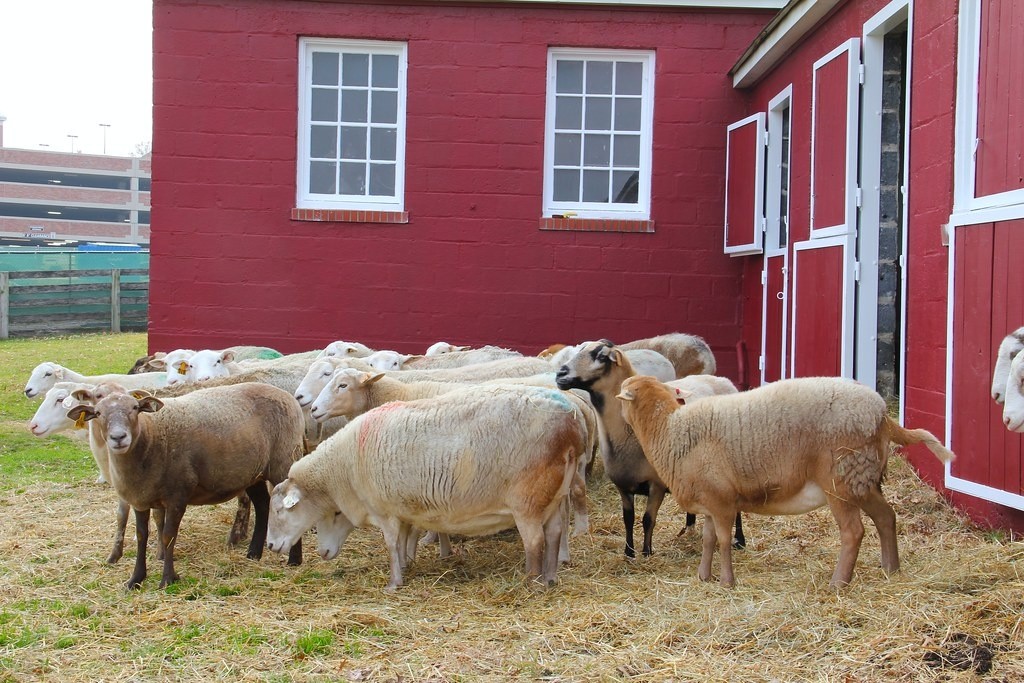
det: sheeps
[22,332,961,593]
[992,324,1024,434]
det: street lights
[67,135,78,152]
[99,124,110,154]
